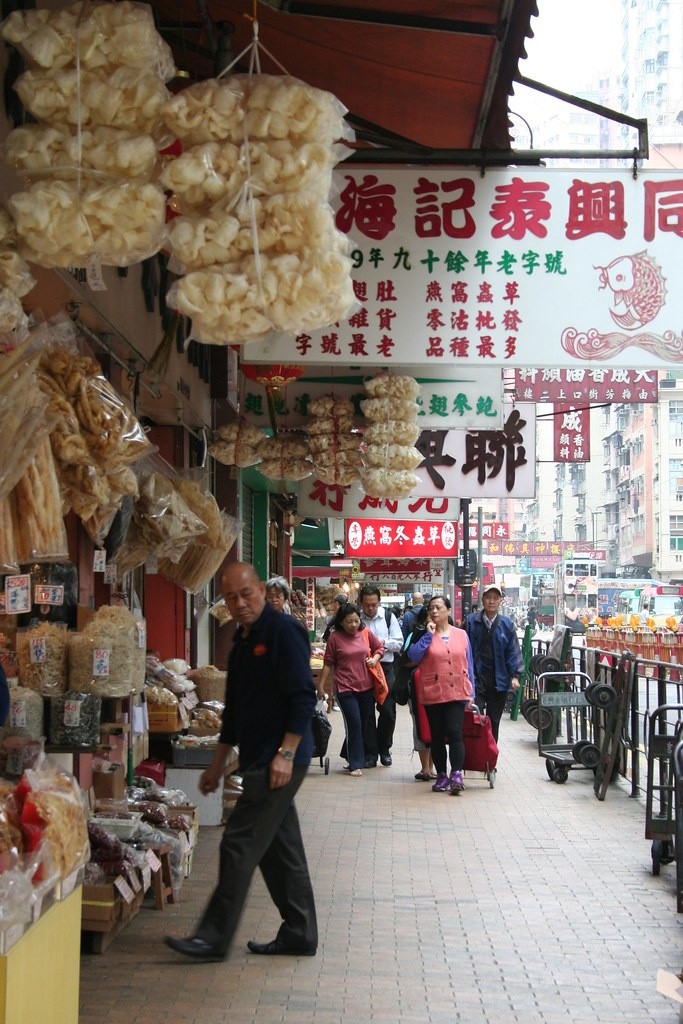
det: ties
[486,620,492,631]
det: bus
[618,585,683,627]
[454,562,495,627]
[554,559,599,635]
[517,572,554,629]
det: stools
[147,843,177,910]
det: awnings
[156,0,539,167]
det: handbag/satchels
[391,674,408,705]
[363,628,389,705]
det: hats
[482,584,503,596]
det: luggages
[459,704,498,790]
[311,708,333,775]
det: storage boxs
[146,703,182,733]
[172,744,216,765]
[168,804,199,876]
[82,887,143,918]
[93,763,126,802]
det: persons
[509,605,536,627]
[401,605,438,780]
[321,596,346,641]
[402,592,425,637]
[267,577,290,613]
[462,585,521,746]
[388,606,412,627]
[359,587,404,768]
[405,596,476,792]
[317,604,384,776]
[161,562,318,959]
[641,603,648,622]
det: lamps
[301,518,318,529]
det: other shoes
[414,771,430,781]
[351,769,362,777]
[163,935,227,961]
[428,771,437,778]
[247,939,314,956]
[378,749,392,766]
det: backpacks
[410,609,421,631]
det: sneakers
[449,770,464,794]
[432,772,450,791]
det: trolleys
[463,703,499,789]
[645,704,683,913]
[504,625,532,720]
[573,651,636,800]
[536,671,616,783]
[521,624,573,745]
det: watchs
[278,747,295,759]
